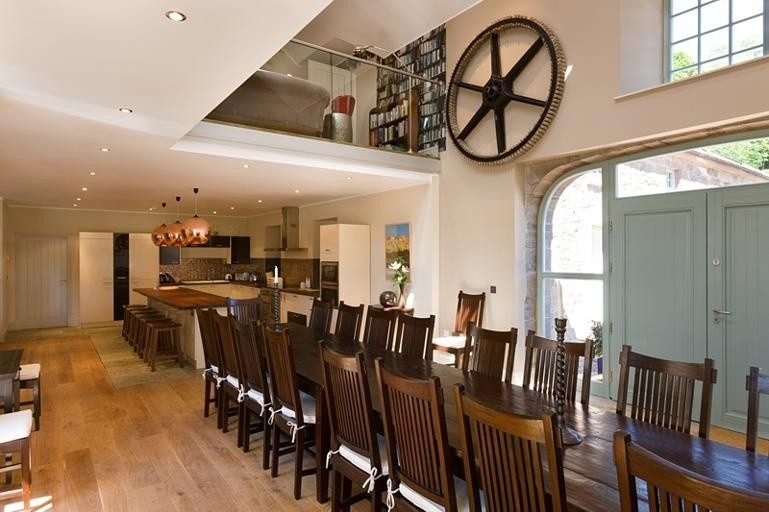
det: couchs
[206,67,332,140]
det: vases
[396,284,406,308]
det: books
[369,23,447,160]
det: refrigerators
[78,231,161,324]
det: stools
[0,407,34,512]
[0,362,42,433]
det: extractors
[264,205,312,253]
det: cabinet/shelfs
[365,88,419,154]
[374,22,446,153]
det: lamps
[151,185,211,248]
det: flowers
[388,261,410,286]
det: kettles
[224,273,233,281]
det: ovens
[320,261,339,306]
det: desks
[0,349,26,417]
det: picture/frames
[382,222,411,285]
